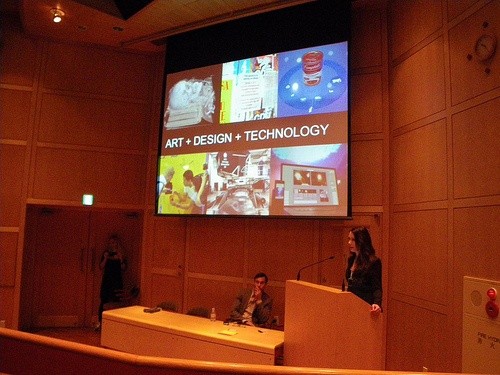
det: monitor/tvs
[280,163,339,207]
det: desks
[101,306,283,366]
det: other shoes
[95,322,101,331]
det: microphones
[297,255,335,280]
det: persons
[158,168,207,213]
[346,226,382,314]
[225,273,272,327]
[94,236,128,332]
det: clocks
[473,34,496,61]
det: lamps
[53,11,62,24]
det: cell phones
[274,181,285,202]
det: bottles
[210,307,217,322]
